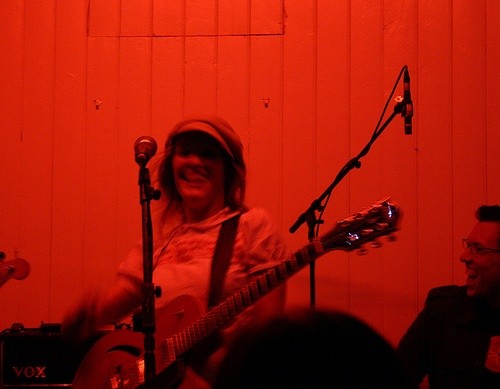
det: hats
[164,115,243,168]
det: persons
[396,204,500,389]
[57,111,285,389]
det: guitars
[0,252,31,288]
[68,200,405,389]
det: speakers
[0,325,116,389]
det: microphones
[133,136,157,164]
[403,69,413,135]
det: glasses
[462,239,500,256]
